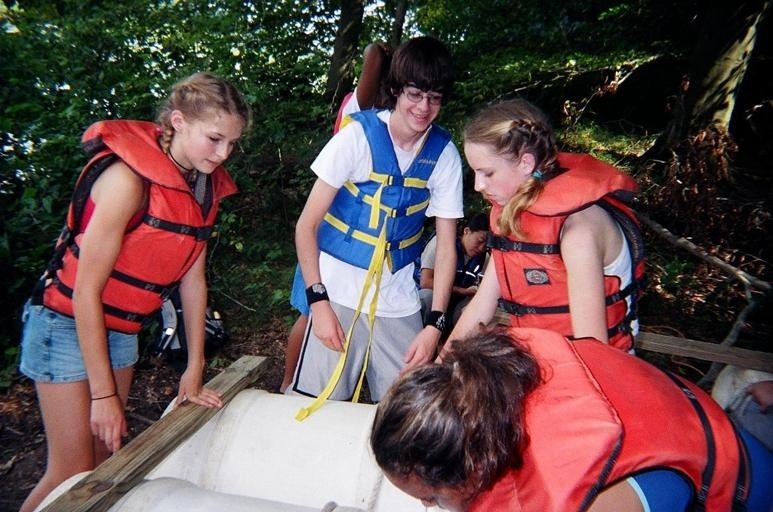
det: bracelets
[306,283,330,305]
[425,311,446,332]
[91,392,118,401]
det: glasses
[402,91,443,105]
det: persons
[435,100,646,367]
[281,39,392,395]
[284,36,466,406]
[710,362,773,451]
[369,327,752,512]
[418,213,491,328]
[17,71,250,512]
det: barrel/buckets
[33,469,370,512]
[143,389,453,512]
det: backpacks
[151,291,225,361]
